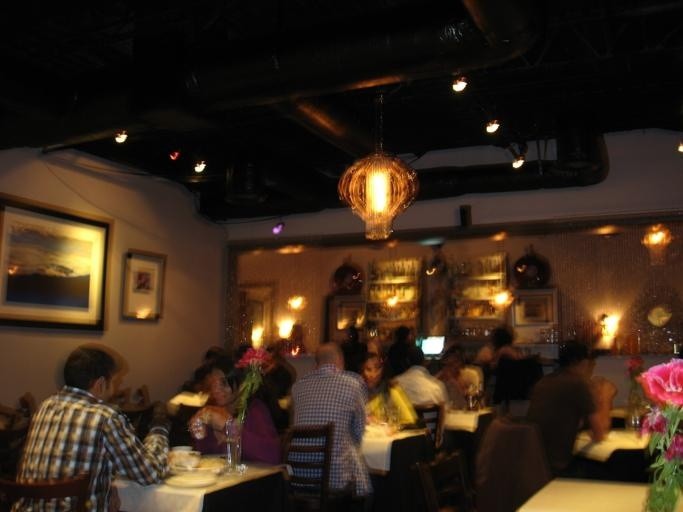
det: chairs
[0,392,37,477]
[0,476,90,512]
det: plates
[163,471,217,489]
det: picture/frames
[122,249,167,321]
[0,193,114,331]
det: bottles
[189,418,208,441]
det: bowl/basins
[167,451,201,468]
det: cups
[225,420,244,475]
[540,326,681,356]
[171,446,193,451]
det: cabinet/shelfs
[324,252,562,376]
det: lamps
[450,71,525,170]
[114,129,206,173]
[639,223,674,265]
[336,89,421,240]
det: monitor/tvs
[415,335,447,360]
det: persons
[165,326,617,511]
[12,342,170,510]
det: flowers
[622,356,645,391]
[634,358,683,512]
[234,347,276,422]
